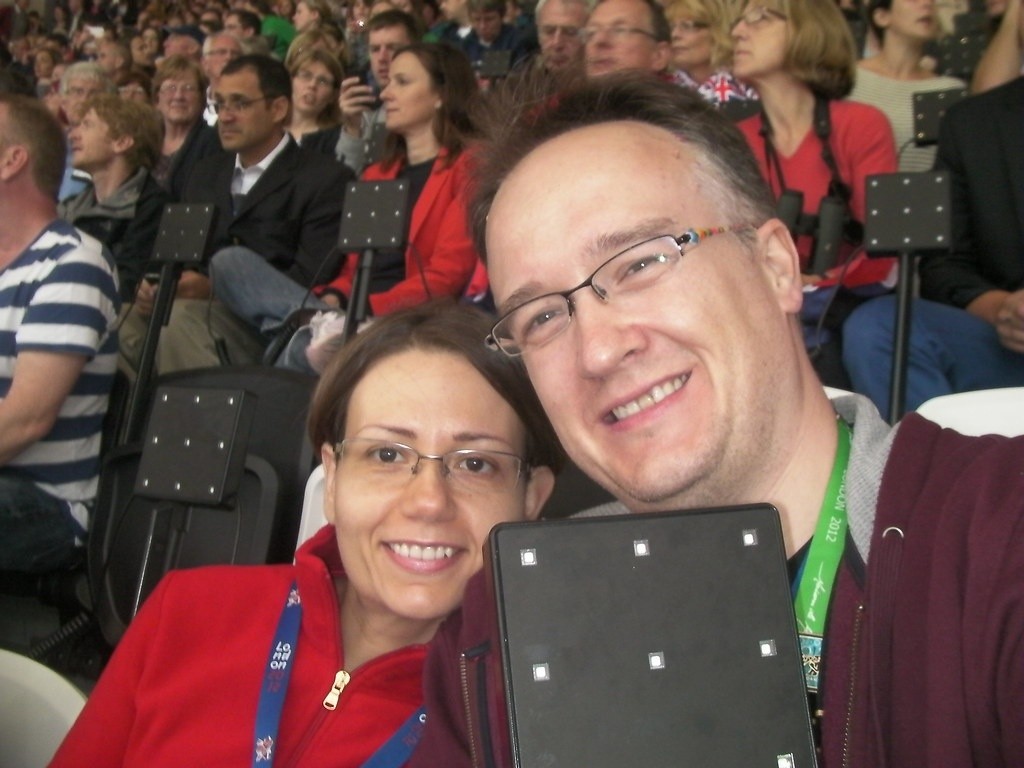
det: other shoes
[305,313,369,378]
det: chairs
[87,365,319,648]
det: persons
[207,43,497,372]
[390,73,1024,768]
[115,56,347,388]
[662,0,760,111]
[729,0,897,388]
[1,0,533,211]
[46,301,557,768]
[842,75,1024,428]
[505,0,598,104]
[59,97,167,302]
[577,0,671,84]
[834,0,1024,78]
[0,94,122,592]
[842,0,968,177]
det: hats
[159,24,203,44]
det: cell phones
[346,67,370,107]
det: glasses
[294,69,332,90]
[160,83,199,99]
[483,219,766,357]
[668,20,712,34]
[728,7,786,29]
[205,48,241,58]
[333,437,534,496]
[207,92,280,112]
[575,24,660,44]
